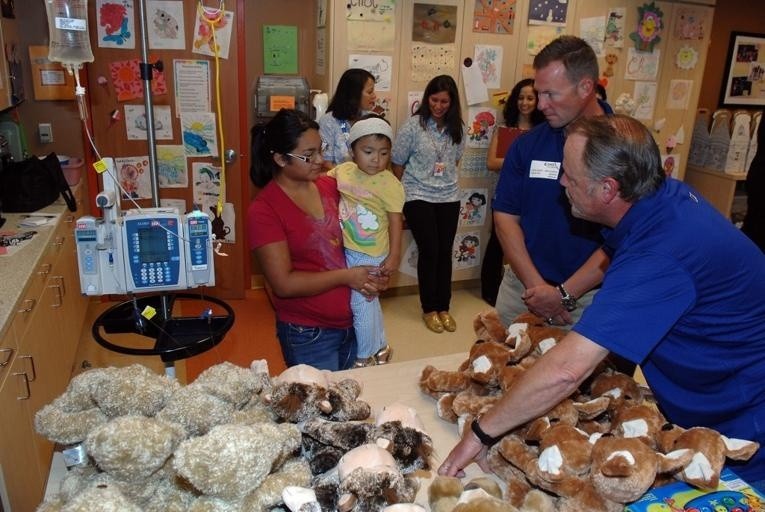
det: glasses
[287,149,324,162]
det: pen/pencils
[21,215,57,219]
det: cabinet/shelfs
[310,0,716,299]
[1,176,91,512]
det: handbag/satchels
[0,152,62,212]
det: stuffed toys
[33,308,760,510]
[35,361,435,512]
[420,312,758,511]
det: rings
[441,463,449,470]
[548,318,554,325]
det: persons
[318,113,406,369]
[434,114,762,492]
[316,68,376,171]
[390,75,466,331]
[482,78,548,306]
[494,34,615,393]
[244,110,391,370]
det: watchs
[472,412,500,448]
[557,284,577,313]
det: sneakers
[422,312,456,332]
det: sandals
[349,344,392,369]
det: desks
[684,162,760,218]
[43,352,765,510]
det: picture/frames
[717,30,764,111]
